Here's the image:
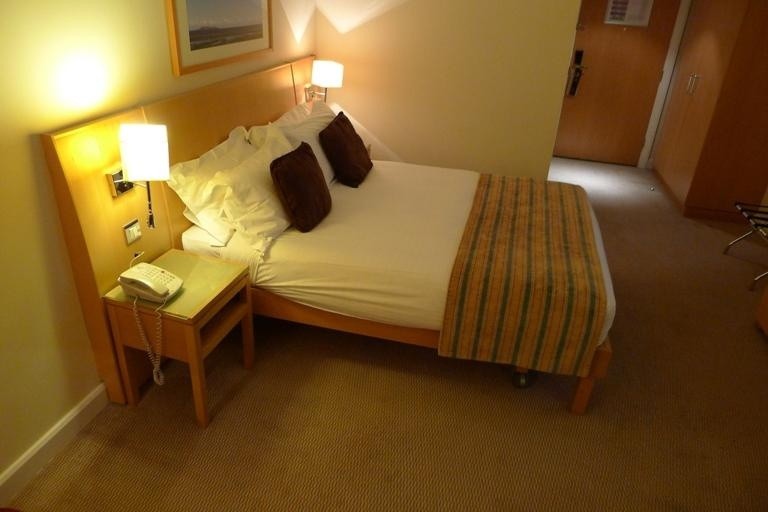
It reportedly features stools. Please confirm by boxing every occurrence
[724,201,768,291]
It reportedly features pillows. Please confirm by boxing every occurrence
[247,100,310,149]
[166,126,262,246]
[318,111,374,188]
[270,141,332,233]
[278,103,370,186]
[201,122,296,255]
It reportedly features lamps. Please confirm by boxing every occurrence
[118,122,170,229]
[311,60,344,102]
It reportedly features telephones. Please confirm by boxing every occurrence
[117,261,185,307]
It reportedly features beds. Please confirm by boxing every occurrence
[40,55,617,415]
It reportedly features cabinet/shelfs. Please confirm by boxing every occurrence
[647,0,768,213]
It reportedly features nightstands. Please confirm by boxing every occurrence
[101,247,256,428]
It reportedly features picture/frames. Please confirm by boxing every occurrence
[164,0,273,79]
